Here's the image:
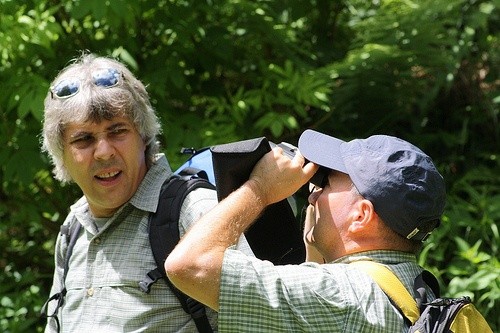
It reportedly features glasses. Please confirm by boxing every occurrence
[49,68,126,99]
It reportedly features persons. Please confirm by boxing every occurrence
[165,129,445,333]
[40,54,257,333]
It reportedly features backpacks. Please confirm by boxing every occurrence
[348,260,494,333]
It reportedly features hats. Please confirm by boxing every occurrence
[298,129,446,244]
